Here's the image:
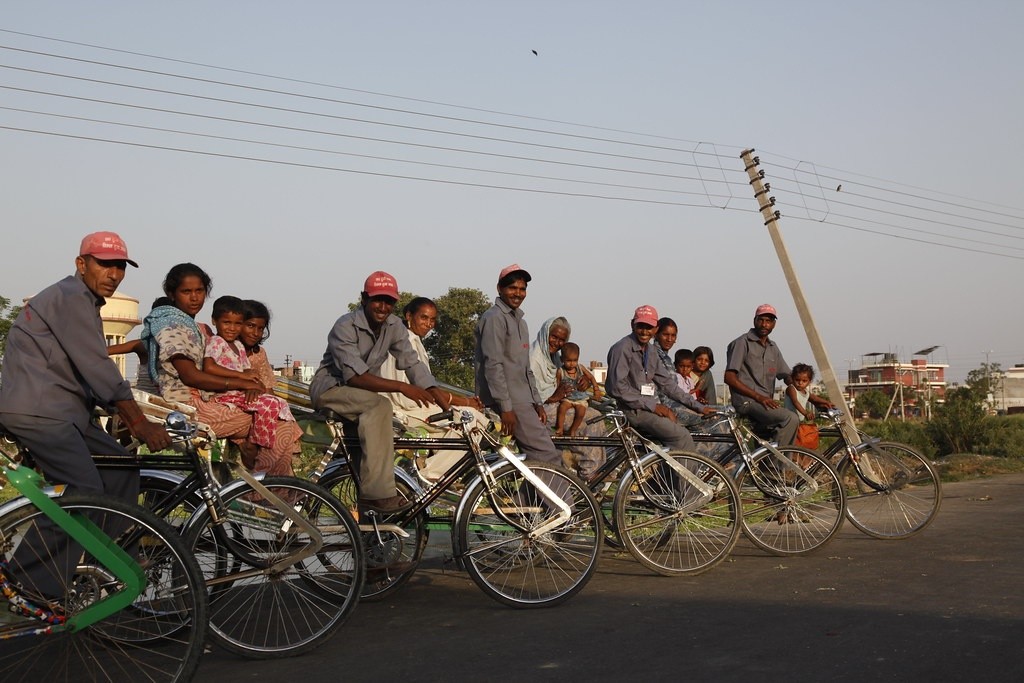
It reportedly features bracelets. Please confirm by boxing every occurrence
[448,393,452,404]
[593,386,600,392]
[464,397,469,405]
[225,377,228,388]
[447,406,453,411]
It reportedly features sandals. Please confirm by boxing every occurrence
[362,555,412,571]
[357,495,414,514]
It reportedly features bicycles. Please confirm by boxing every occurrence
[0,448,209,683]
[0,396,943,664]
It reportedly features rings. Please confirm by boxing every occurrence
[565,394,566,397]
[160,440,167,443]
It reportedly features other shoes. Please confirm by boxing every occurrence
[777,507,815,525]
[760,466,793,484]
[558,508,593,542]
[9,588,67,625]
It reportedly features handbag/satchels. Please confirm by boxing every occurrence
[793,419,819,450]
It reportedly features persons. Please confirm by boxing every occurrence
[474,264,622,532]
[605,305,717,516]
[0,230,304,622]
[309,271,462,584]
[724,304,835,525]
[377,297,489,492]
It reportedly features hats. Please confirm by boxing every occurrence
[756,303,778,321]
[80,232,139,269]
[364,271,401,301]
[498,264,532,282]
[633,305,659,327]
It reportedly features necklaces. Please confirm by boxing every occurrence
[566,369,577,378]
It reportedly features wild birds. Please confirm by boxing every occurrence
[532,50,537,56]
[837,185,842,192]
